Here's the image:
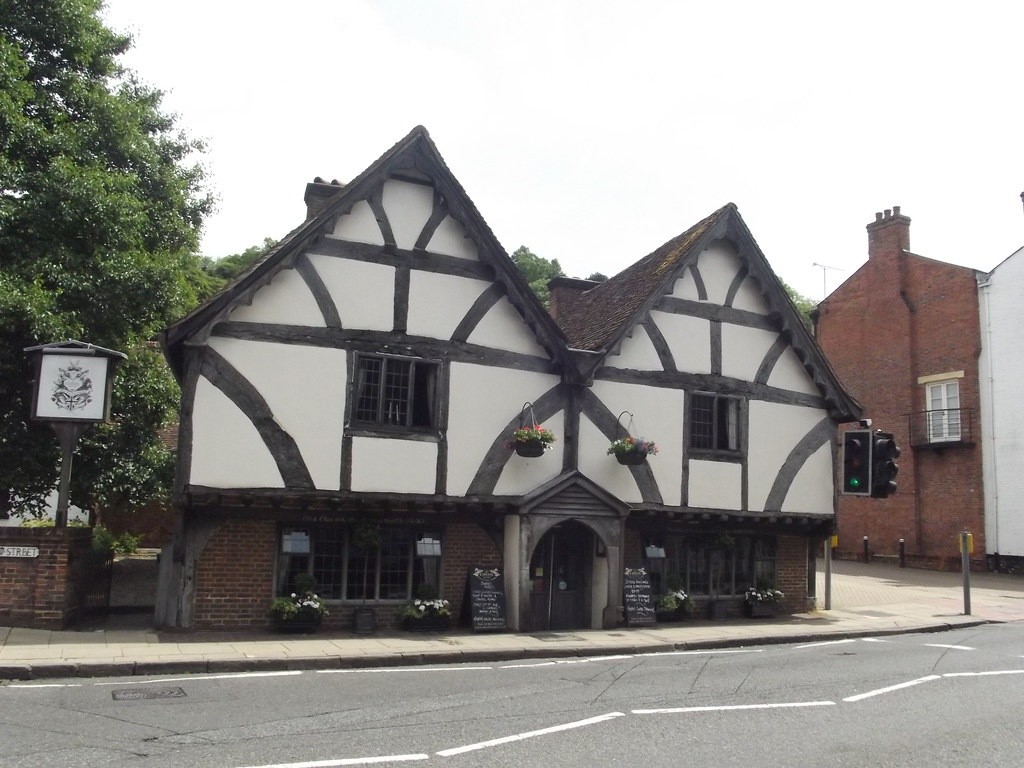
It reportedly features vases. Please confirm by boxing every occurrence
[750,603,779,616]
[613,450,647,465]
[273,617,321,632]
[405,618,449,632]
[515,438,547,457]
[657,612,684,622]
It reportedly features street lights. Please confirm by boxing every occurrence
[812,262,842,301]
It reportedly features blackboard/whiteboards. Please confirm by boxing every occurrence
[468,563,508,632]
[623,568,656,628]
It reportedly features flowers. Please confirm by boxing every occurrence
[268,592,330,618]
[745,587,785,606]
[606,437,659,457]
[505,424,556,451]
[653,589,693,612]
[400,599,452,619]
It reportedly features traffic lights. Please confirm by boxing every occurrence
[842,430,873,496]
[874,433,901,502]
